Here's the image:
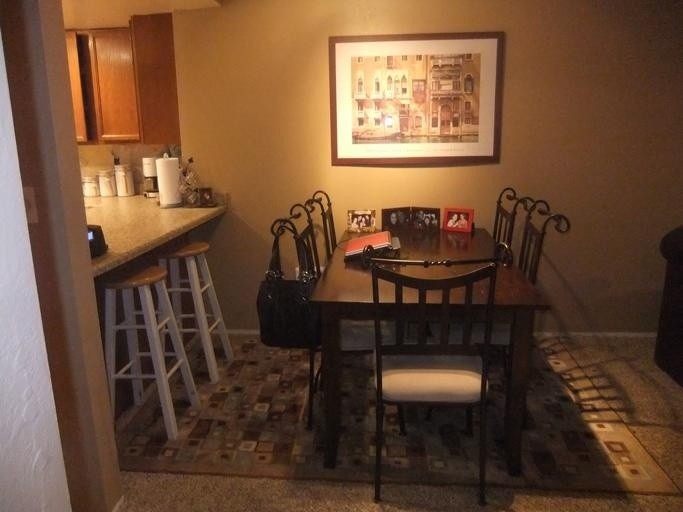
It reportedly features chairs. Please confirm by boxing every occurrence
[408,200,570,419]
[493,188,536,247]
[362,240,512,507]
[271,204,406,431]
[305,190,337,261]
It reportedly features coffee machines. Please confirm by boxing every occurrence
[142,157,160,198]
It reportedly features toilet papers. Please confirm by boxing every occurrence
[154,156,182,206]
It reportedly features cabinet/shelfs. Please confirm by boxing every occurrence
[64,13,181,143]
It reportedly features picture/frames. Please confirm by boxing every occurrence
[382,206,410,237]
[329,31,503,165]
[411,207,440,234]
[443,207,475,233]
[346,209,376,233]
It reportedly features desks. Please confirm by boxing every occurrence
[310,229,552,476]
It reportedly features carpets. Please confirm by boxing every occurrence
[114,334,683,512]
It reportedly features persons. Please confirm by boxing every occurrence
[414,210,438,232]
[447,213,468,228]
[384,211,409,228]
[351,215,371,228]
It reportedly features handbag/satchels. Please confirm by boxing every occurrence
[257,273,322,352]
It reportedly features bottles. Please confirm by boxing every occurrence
[82,163,135,197]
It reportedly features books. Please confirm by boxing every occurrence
[345,230,393,256]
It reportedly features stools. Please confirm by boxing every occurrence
[158,241,234,383]
[104,265,203,441]
